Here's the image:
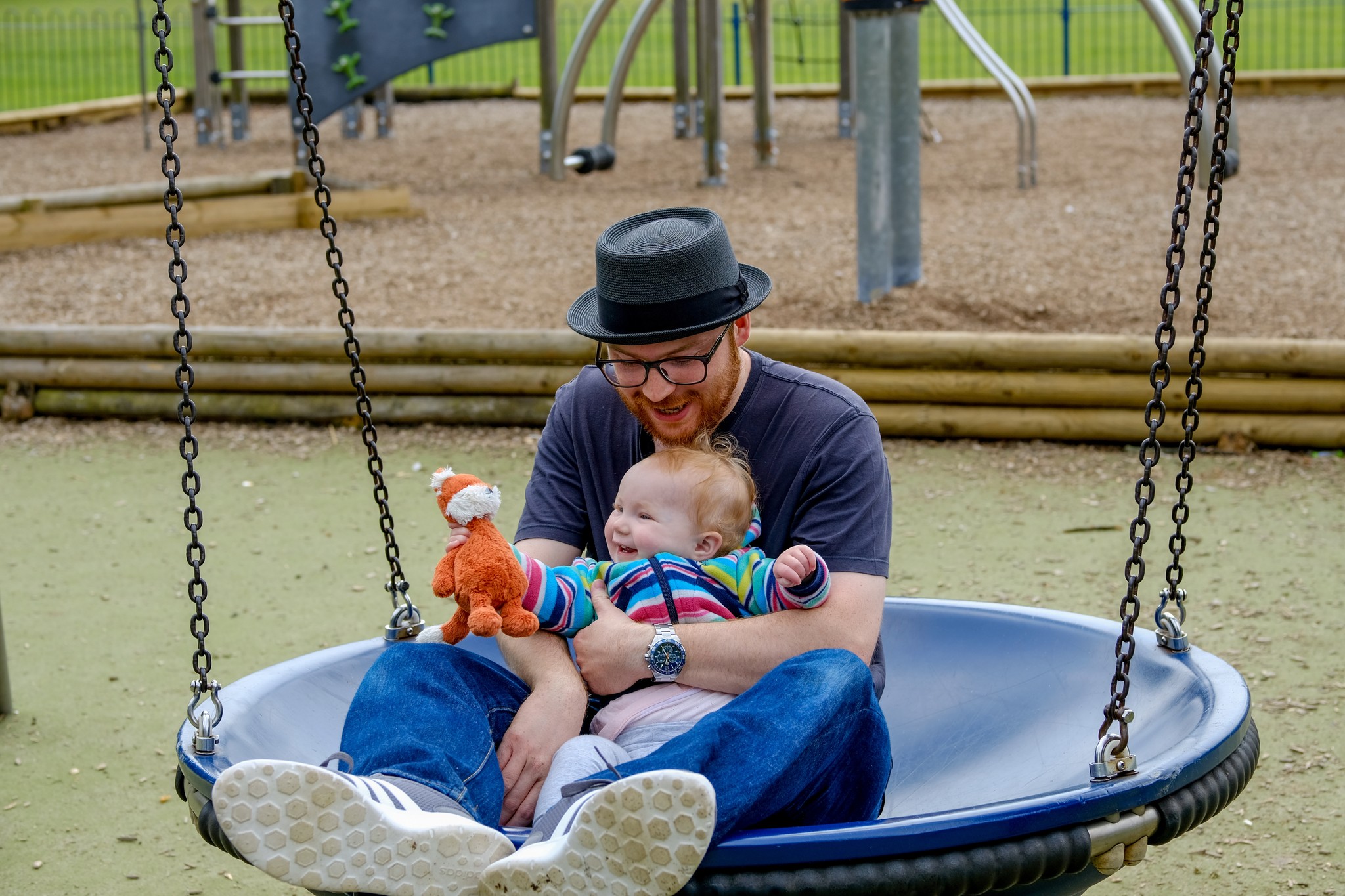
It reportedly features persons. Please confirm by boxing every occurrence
[212,222,896,896]
[444,423,831,835]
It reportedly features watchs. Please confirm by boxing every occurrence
[643,623,686,682]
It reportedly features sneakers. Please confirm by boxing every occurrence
[211,751,516,896]
[478,746,718,896]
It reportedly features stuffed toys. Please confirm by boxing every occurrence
[414,464,540,645]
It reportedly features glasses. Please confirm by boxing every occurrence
[594,320,733,388]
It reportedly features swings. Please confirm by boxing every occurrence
[138,0,1261,894]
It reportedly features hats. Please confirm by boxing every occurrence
[566,208,772,345]
